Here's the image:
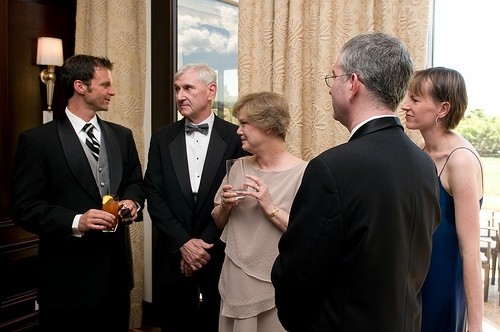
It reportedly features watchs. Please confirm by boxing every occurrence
[267,206,280,219]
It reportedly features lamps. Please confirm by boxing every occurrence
[36,37,64,124]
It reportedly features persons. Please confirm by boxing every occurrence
[271,32,441,332]
[18,54,145,332]
[402,65,485,332]
[211,91,310,331]
[144,64,253,331]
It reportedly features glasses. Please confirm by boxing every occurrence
[324,74,352,87]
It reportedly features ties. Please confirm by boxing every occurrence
[82,123,100,161]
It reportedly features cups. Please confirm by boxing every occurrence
[102,194,118,232]
[226,159,248,203]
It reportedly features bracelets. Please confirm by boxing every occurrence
[133,200,141,213]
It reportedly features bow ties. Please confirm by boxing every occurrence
[184,122,209,136]
